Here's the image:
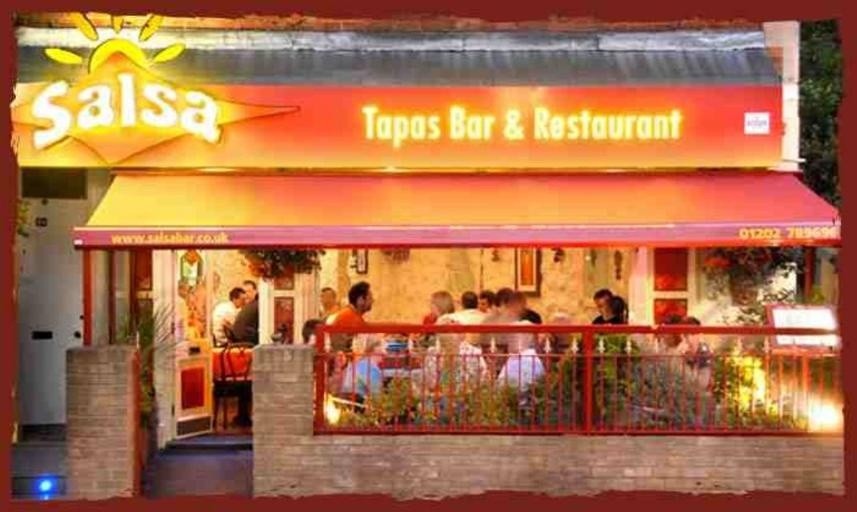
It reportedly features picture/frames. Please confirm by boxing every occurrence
[513,246,540,297]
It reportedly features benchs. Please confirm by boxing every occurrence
[762,301,841,397]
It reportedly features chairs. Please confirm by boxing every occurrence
[212,342,253,429]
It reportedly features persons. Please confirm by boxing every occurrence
[211,281,258,424]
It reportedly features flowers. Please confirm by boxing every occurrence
[700,246,807,302]
[242,247,328,282]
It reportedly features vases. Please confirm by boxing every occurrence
[248,262,271,277]
[729,278,758,305]
